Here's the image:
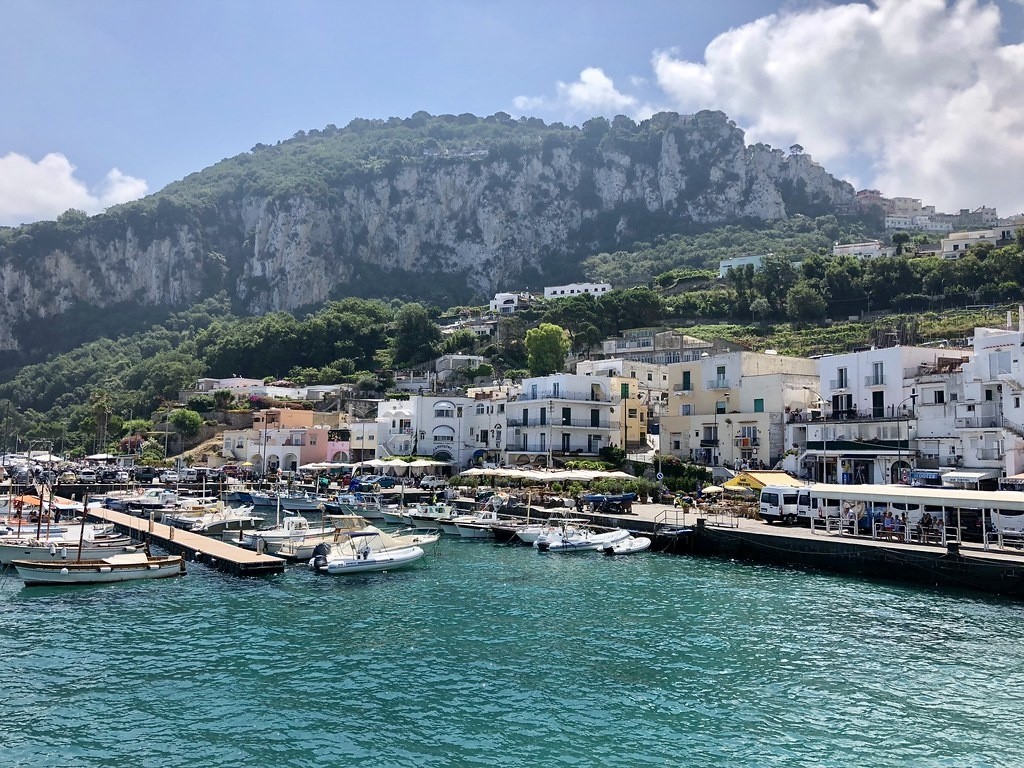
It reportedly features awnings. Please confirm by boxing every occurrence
[940,467,1002,483]
[1000,472,1024,490]
[910,471,939,479]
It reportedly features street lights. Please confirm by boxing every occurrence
[128,407,133,453]
[103,411,109,453]
[162,403,169,464]
[351,406,378,477]
[550,418,567,470]
[803,385,845,484]
[714,393,730,467]
[897,393,919,483]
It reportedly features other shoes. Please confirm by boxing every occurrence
[888,538,893,541]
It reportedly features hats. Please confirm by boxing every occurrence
[882,512,887,514]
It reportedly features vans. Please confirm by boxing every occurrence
[177,468,198,483]
[796,485,840,530]
[841,473,1024,549]
[217,464,239,478]
[758,483,800,526]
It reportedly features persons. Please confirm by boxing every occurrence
[878,511,944,546]
[733,457,765,475]
[14,509,62,523]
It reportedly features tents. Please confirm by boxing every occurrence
[297,459,454,479]
[719,470,805,489]
[459,468,637,491]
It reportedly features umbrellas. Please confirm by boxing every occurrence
[242,461,255,472]
[723,485,746,503]
[701,485,725,497]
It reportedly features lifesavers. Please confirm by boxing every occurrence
[138,488,144,495]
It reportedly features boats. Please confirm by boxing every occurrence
[0,458,592,549]
[313,530,426,576]
[10,487,183,587]
[536,528,631,555]
[604,536,651,555]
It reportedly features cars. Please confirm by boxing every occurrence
[36,471,57,484]
[116,471,131,484]
[95,469,117,484]
[74,470,96,484]
[11,470,34,485]
[58,471,77,485]
[159,470,179,483]
[366,475,396,489]
[360,474,381,483]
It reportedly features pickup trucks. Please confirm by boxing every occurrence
[133,466,155,484]
[419,476,446,491]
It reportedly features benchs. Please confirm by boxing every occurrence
[986,530,1024,550]
[812,517,959,546]
